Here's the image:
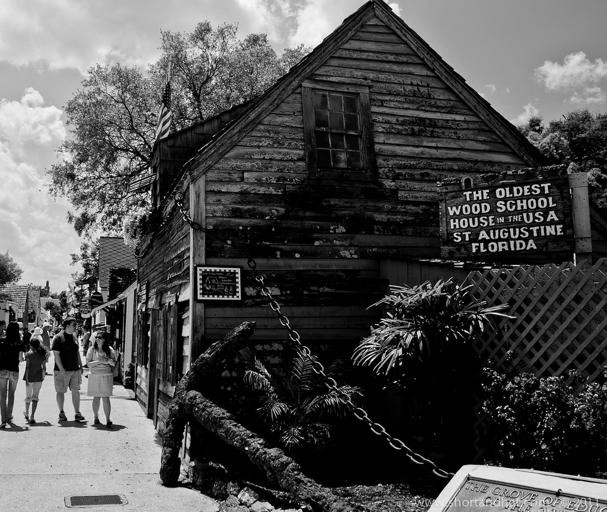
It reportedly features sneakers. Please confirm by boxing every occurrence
[75,412,85,420]
[0,412,36,428]
[59,411,67,421]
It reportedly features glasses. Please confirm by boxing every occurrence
[95,336,102,338]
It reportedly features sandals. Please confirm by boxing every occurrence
[94,419,114,429]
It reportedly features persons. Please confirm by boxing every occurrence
[0,319,117,431]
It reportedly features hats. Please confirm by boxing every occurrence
[42,322,52,331]
[35,327,43,334]
